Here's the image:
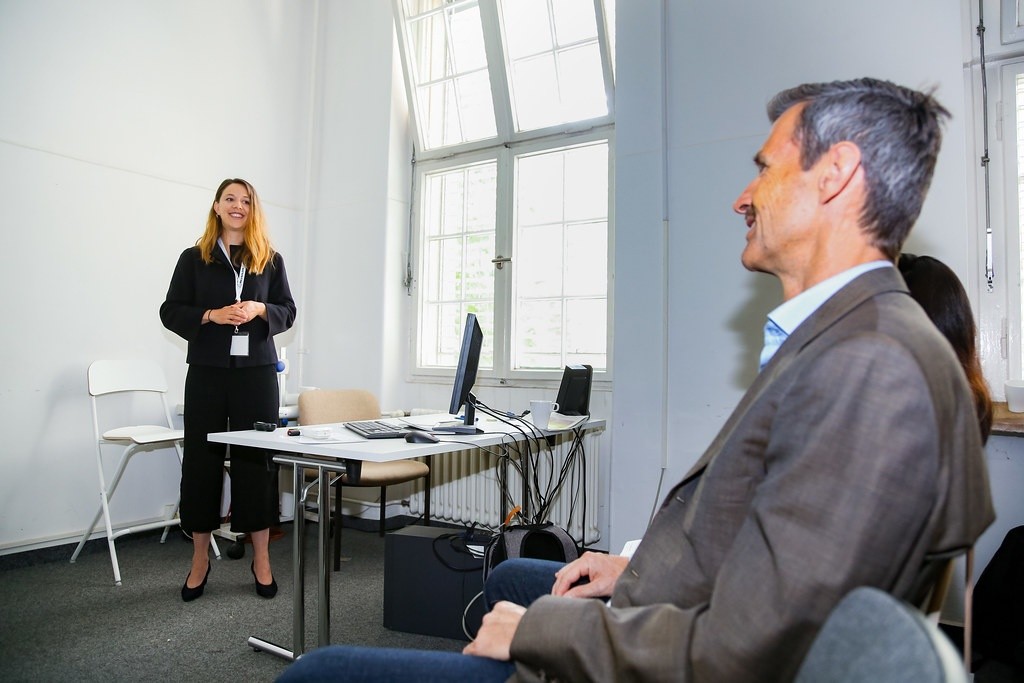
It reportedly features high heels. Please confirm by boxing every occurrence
[182,557,211,602]
[250,560,279,599]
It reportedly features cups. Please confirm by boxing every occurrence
[1004,379,1024,413]
[530,400,559,431]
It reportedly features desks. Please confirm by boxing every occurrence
[205,402,613,661]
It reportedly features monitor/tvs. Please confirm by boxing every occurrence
[432,313,484,434]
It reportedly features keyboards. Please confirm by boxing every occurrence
[344,421,412,440]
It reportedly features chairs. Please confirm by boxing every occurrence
[297,388,437,576]
[67,355,222,586]
[749,585,986,683]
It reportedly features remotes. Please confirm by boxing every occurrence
[253,421,276,431]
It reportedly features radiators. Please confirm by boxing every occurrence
[409,408,601,545]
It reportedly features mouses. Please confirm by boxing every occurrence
[405,431,440,443]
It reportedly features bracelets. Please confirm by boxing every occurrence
[208,308,213,322]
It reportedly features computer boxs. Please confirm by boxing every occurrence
[382,525,504,641]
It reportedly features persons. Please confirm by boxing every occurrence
[274,78,999,683]
[898,253,994,448]
[159,178,298,603]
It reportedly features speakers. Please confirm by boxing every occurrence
[554,364,594,417]
